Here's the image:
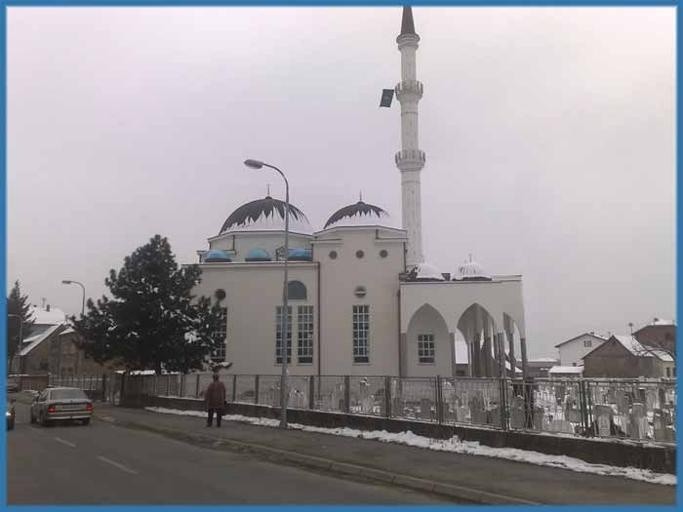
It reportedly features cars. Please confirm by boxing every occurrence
[6,396,15,430]
[30,385,94,428]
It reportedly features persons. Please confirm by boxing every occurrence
[203,372,227,428]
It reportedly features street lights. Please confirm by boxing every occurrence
[244,159,289,425]
[8,312,22,390]
[64,280,84,377]
